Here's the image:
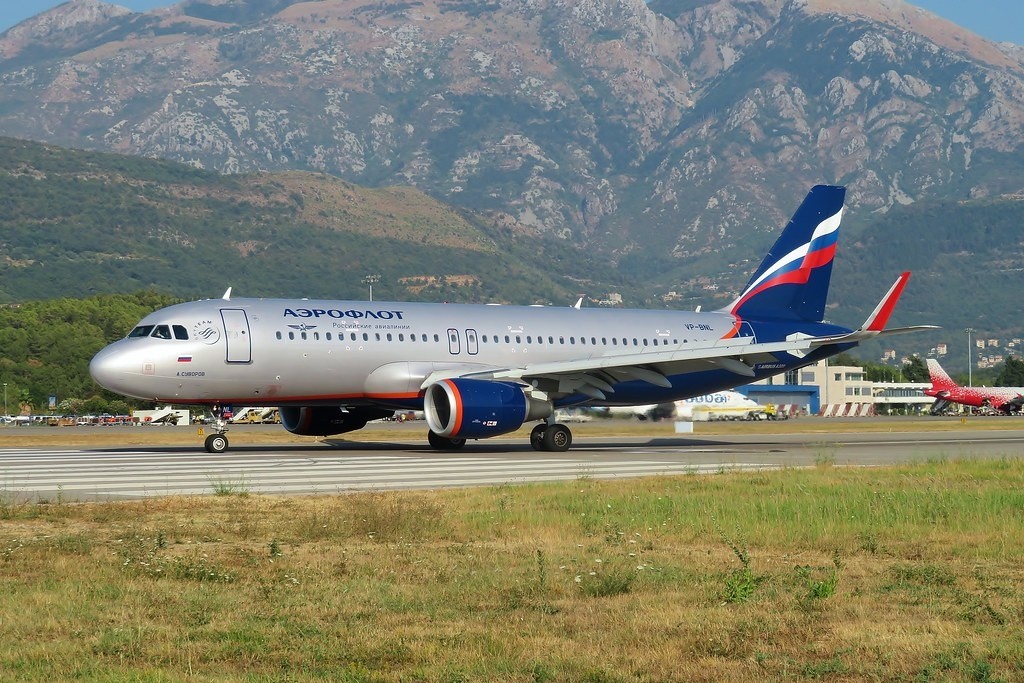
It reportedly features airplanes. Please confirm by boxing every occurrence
[90,185,942,451]
[924,359,1024,416]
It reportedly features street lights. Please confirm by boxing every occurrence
[3,382,8,416]
[362,273,381,301]
[963,328,977,415]
